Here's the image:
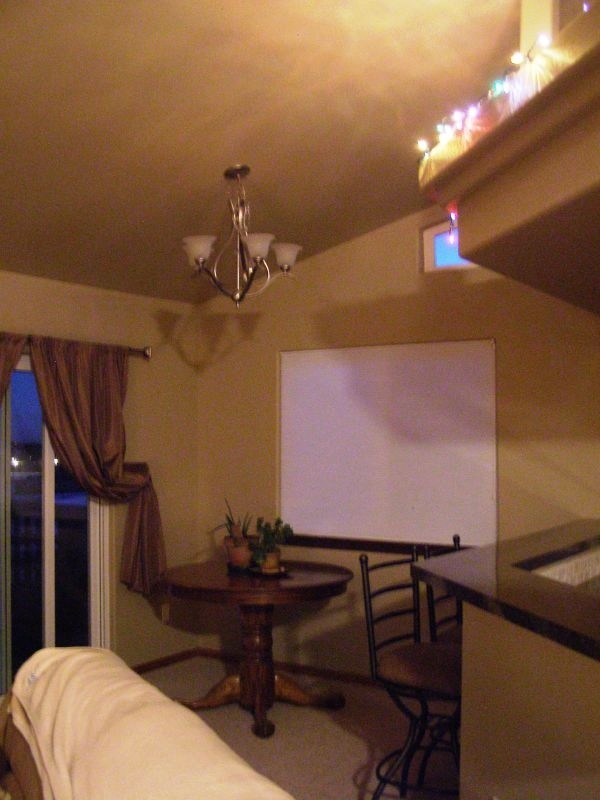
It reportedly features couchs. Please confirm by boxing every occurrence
[0,647,294,800]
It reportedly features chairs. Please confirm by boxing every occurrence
[359,534,462,800]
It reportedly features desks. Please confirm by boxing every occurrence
[166,560,355,738]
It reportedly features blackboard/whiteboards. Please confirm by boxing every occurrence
[279,337,498,548]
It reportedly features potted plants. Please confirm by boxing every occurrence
[209,498,294,576]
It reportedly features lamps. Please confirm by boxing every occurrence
[182,163,302,310]
